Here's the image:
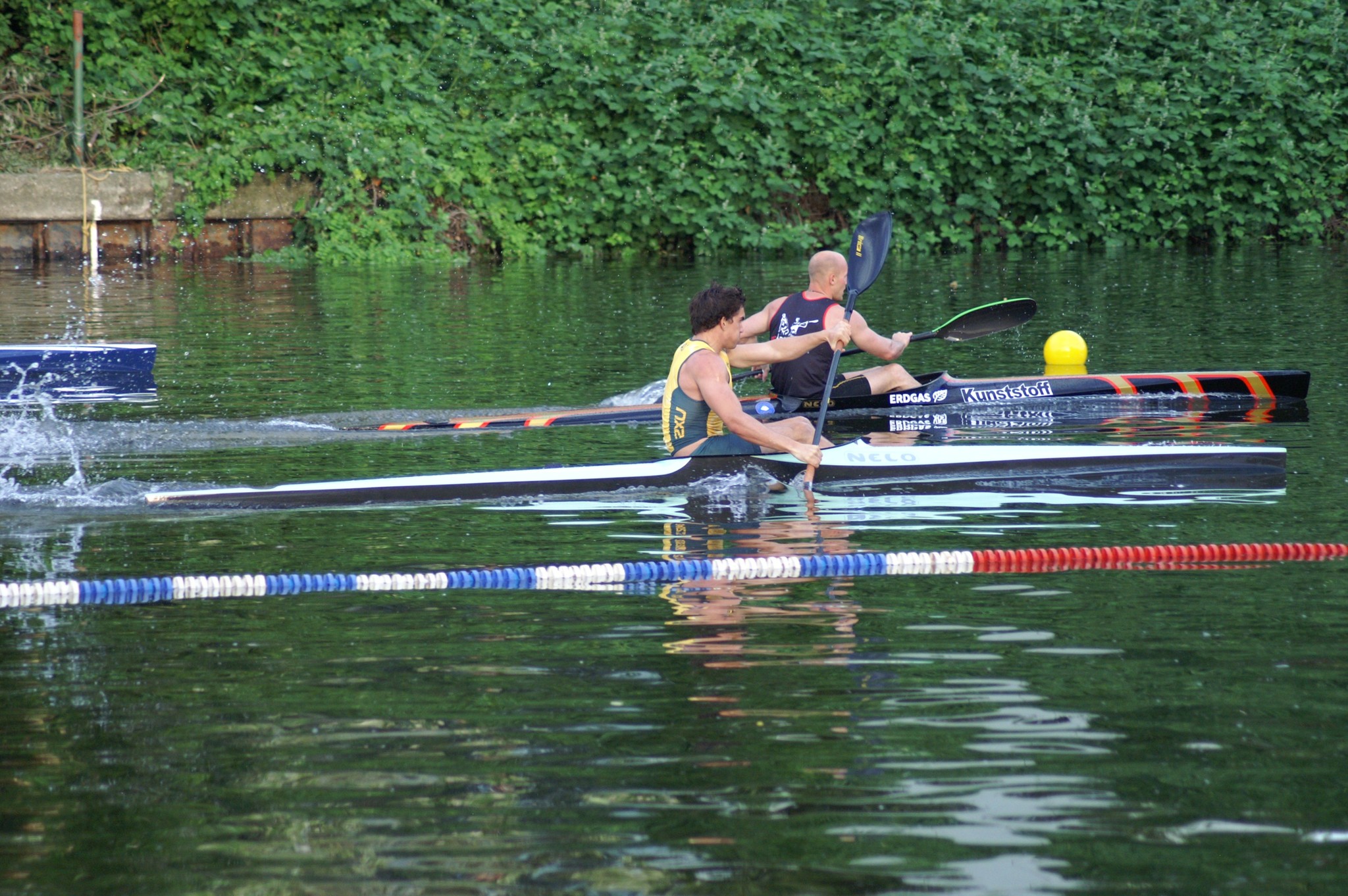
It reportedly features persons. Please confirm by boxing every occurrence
[733,250,925,401]
[663,280,833,467]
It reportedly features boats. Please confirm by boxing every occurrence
[144,443,1288,505]
[0,342,160,382]
[340,365,1311,433]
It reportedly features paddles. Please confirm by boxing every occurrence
[729,297,1038,382]
[800,207,895,500]
